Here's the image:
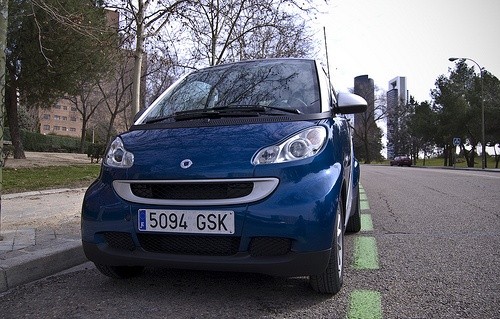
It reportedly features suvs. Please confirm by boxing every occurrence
[390,156,412,167]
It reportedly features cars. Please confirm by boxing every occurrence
[80,57,368,295]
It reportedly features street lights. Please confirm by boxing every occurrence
[449,57,485,169]
